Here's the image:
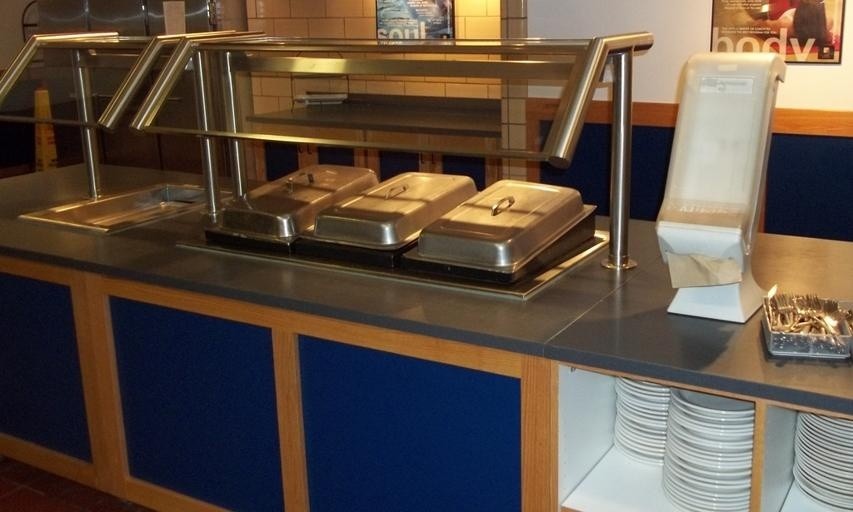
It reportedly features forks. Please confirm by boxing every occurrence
[768,284,850,348]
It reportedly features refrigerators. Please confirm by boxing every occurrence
[37,0,258,180]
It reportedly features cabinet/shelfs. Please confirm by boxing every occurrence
[536,357,853,510]
[366,129,488,193]
[0,246,109,494]
[79,272,560,510]
[251,119,366,184]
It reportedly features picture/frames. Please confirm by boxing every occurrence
[709,0,845,65]
[375,0,456,40]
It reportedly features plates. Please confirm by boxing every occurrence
[792,411,853,510]
[614,375,755,512]
